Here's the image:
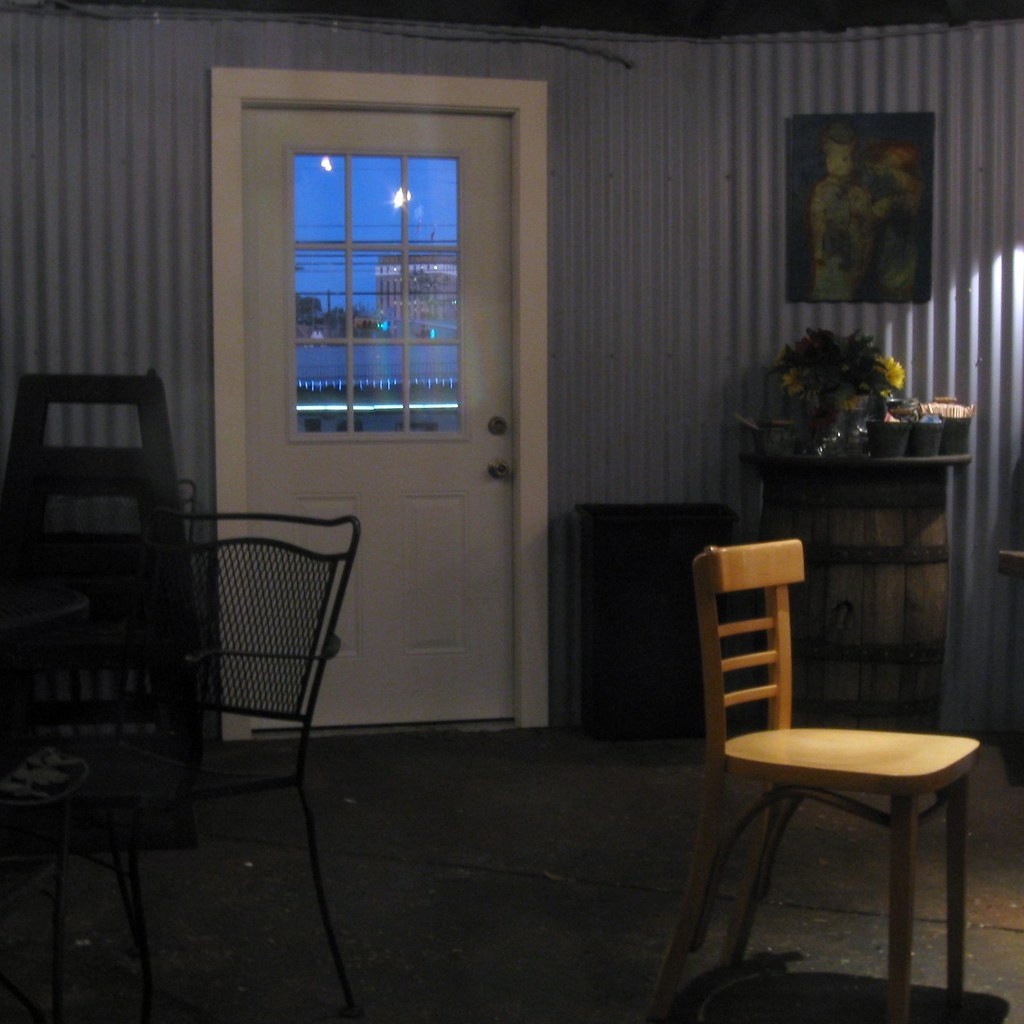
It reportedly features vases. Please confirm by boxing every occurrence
[803,395,871,455]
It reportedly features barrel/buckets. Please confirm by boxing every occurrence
[758,477,951,733]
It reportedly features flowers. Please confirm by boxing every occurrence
[769,327,907,407]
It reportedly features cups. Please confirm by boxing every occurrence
[941,417,970,455]
[910,424,945,457]
[864,421,909,459]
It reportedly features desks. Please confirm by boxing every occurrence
[738,453,972,728]
[0,579,92,773]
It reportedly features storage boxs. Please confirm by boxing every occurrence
[577,501,740,741]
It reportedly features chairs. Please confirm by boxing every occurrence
[0,509,361,1024]
[637,537,981,1024]
[0,370,199,801]
